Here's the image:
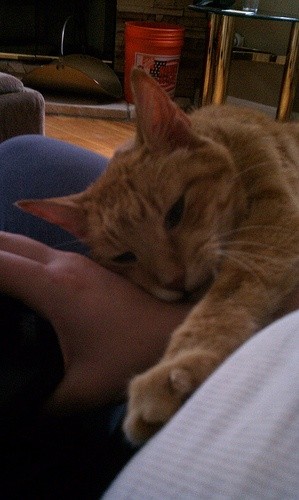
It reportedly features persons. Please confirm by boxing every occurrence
[0,135,299,500]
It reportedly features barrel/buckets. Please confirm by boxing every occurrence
[123,22,183,104]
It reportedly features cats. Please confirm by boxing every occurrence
[14,66,299,448]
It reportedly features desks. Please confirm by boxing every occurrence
[189,4,299,121]
